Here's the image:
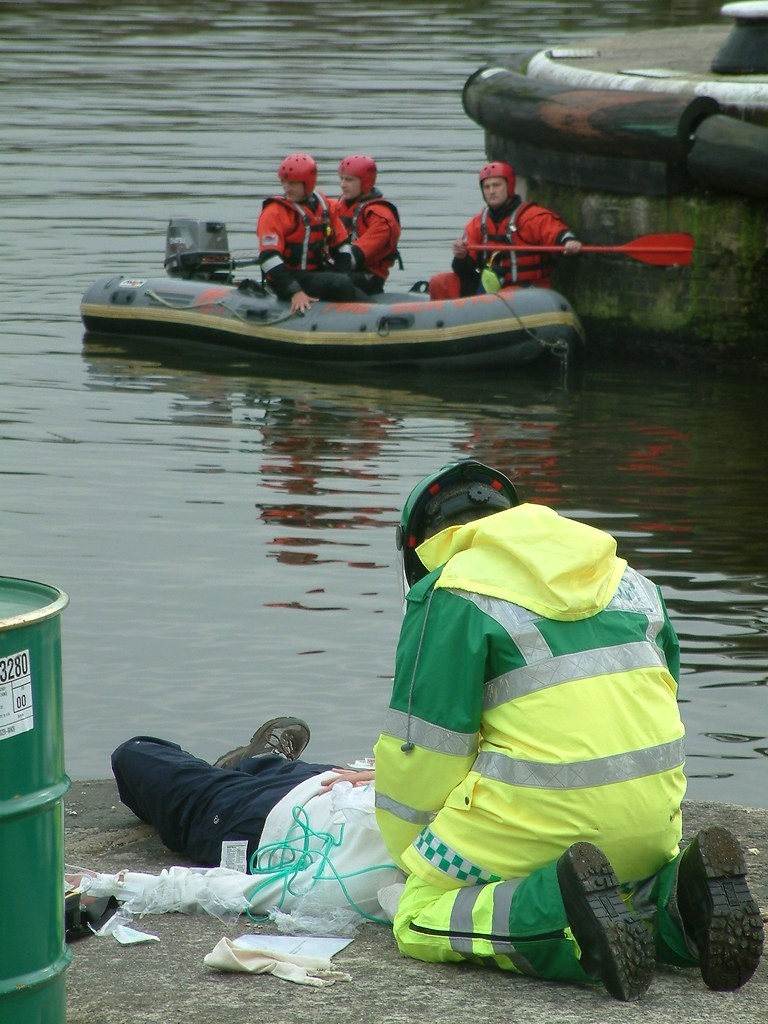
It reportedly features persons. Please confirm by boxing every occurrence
[65,715,406,923]
[257,153,401,315]
[375,456,764,1002]
[429,162,582,300]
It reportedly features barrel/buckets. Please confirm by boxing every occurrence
[0,575,74,1024]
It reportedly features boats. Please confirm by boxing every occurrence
[76,217,588,372]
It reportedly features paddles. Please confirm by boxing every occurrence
[465,233,693,267]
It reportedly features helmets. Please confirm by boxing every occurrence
[480,162,516,204]
[395,458,520,589]
[339,155,377,193]
[278,154,316,195]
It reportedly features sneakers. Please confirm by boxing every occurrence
[212,716,310,771]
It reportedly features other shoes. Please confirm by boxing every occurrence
[556,841,655,1001]
[678,828,764,992]
